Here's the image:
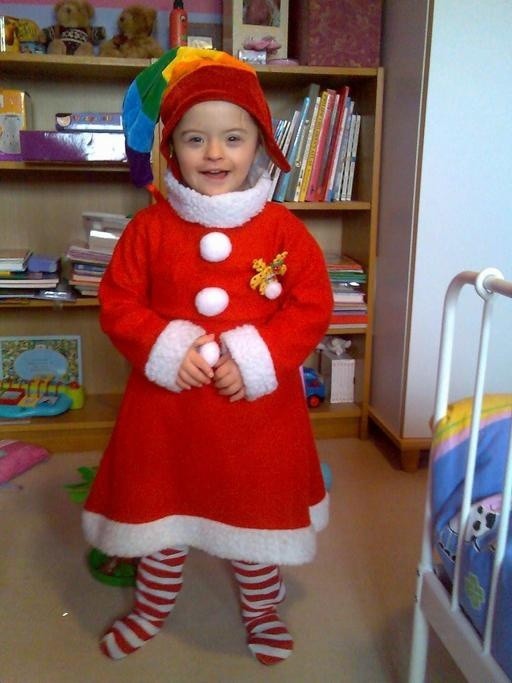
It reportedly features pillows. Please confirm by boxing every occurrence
[0,438,49,484]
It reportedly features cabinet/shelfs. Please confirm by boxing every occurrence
[369,1,512,474]
[0,49,384,455]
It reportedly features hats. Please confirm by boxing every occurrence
[122,46,290,187]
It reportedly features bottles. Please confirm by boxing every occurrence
[169,0,188,51]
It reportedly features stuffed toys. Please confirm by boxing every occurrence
[38,0,105,57]
[100,4,165,58]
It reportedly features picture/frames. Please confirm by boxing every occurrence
[0,335,83,385]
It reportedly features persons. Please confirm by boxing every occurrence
[79,46,333,667]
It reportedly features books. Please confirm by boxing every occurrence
[0,246,78,303]
[319,254,368,330]
[64,210,135,296]
[259,83,360,203]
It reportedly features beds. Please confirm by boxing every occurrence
[408,266,512,683]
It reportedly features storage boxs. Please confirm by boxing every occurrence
[0,88,31,159]
[321,352,355,404]
[290,0,382,67]
[19,129,129,164]
